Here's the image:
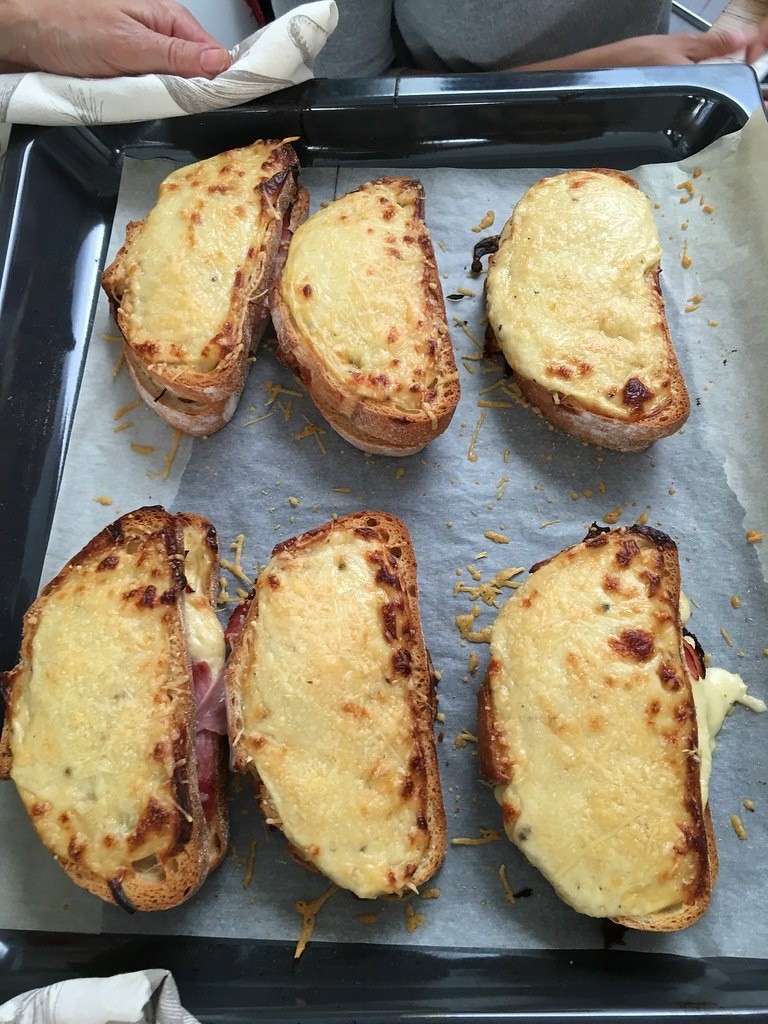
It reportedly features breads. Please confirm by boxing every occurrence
[100,137,310,437]
[0,502,232,914]
[483,523,718,933]
[488,167,692,454]
[268,176,461,457]
[220,510,445,902]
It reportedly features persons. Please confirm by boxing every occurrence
[0,0,768,79]
